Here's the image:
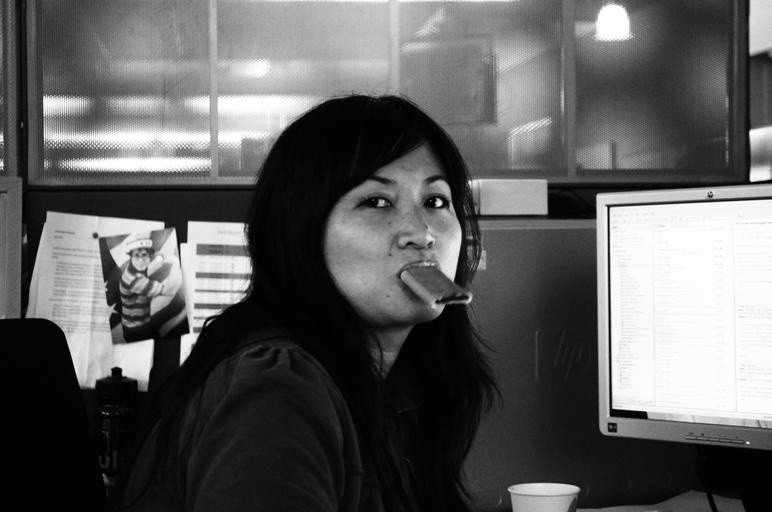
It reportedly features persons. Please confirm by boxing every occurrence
[120,88,509,508]
[116,237,181,343]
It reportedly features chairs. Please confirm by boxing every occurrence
[1,318,82,512]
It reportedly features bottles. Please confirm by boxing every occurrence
[94,368,139,507]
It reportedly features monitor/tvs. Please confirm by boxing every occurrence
[596,184,772,451]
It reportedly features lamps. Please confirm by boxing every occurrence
[594,1,632,42]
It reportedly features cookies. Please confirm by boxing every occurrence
[400,266,473,309]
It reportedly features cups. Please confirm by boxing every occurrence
[507,483,579,512]
